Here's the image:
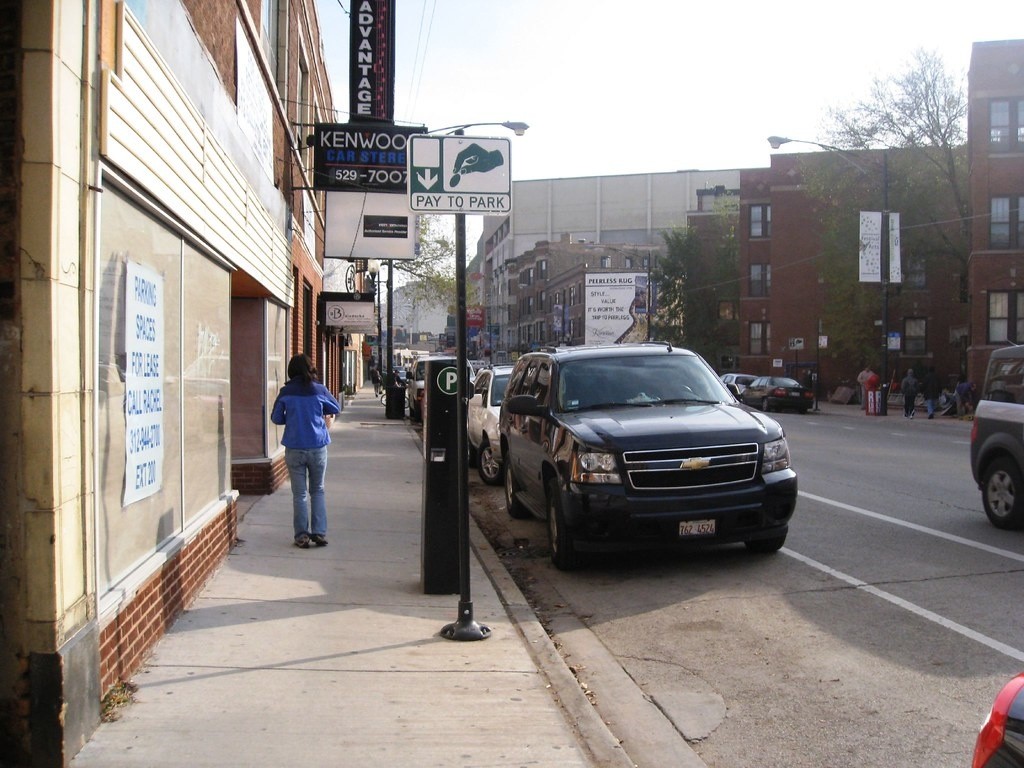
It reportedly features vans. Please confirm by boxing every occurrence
[407,355,474,424]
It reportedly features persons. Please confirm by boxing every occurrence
[806,369,813,388]
[953,375,968,417]
[394,369,408,387]
[271,354,340,548]
[857,365,875,410]
[372,371,383,398]
[922,368,939,419]
[901,369,919,419]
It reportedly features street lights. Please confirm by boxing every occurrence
[441,126,528,643]
[585,243,652,341]
[368,258,381,396]
[768,134,892,416]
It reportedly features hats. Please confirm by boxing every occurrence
[908,369,914,374]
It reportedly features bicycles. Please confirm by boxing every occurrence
[381,382,411,410]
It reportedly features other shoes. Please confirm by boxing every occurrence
[903,414,913,419]
[928,413,934,419]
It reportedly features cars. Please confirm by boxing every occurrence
[468,362,515,487]
[741,375,814,415]
[719,373,759,402]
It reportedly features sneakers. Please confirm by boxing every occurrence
[294,535,329,549]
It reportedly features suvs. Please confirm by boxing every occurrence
[499,341,797,574]
[969,343,1024,530]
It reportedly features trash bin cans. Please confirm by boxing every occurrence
[864,376,888,416]
[386,387,406,419]
